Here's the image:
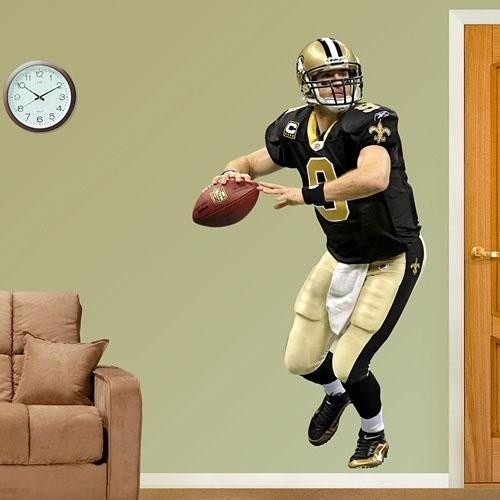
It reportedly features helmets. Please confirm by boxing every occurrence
[296,38,363,113]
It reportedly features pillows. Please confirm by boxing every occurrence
[13,331,109,406]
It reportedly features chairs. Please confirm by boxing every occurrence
[0,291,140,498]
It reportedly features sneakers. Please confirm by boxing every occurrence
[348,429,389,468]
[308,391,351,446]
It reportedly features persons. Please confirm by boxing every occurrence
[212,37,424,468]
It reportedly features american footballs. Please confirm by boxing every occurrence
[193,179,260,227]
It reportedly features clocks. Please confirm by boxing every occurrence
[5,60,77,131]
